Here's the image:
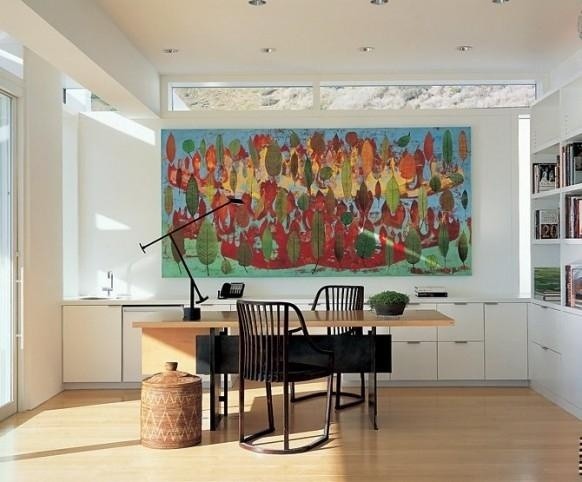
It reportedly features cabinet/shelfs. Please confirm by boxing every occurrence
[62,300,184,390]
[529,299,582,422]
[437,301,485,386]
[529,71,581,310]
[390,302,437,387]
[486,299,529,387]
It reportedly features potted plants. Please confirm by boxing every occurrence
[367,291,409,316]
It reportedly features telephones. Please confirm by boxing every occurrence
[217,282,245,299]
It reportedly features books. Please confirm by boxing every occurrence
[562,142,582,187]
[534,266,561,293]
[564,193,582,239]
[532,154,561,194]
[533,208,561,240]
[414,286,448,297]
[565,264,582,309]
[533,293,561,301]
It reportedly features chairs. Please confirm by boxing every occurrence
[234,300,332,454]
[291,285,365,410]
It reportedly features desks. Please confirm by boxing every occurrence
[131,310,454,431]
